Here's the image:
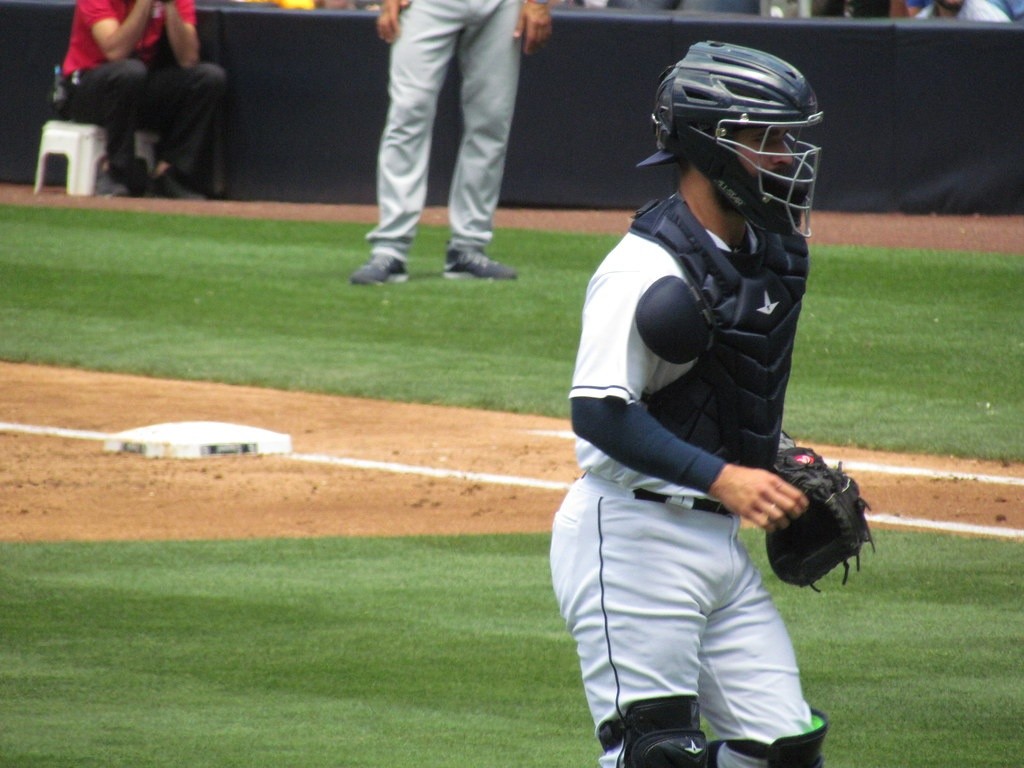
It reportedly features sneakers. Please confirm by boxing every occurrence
[444,251,516,280]
[352,253,407,285]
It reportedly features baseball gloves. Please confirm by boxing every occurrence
[764,446,871,590]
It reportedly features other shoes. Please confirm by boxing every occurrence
[149,166,204,199]
[112,177,130,195]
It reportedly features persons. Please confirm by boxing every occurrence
[549,42,829,768]
[60,0,226,201]
[350,0,553,285]
[915,0,1014,23]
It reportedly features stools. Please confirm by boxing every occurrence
[30,120,159,197]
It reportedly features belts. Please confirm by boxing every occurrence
[635,488,729,516]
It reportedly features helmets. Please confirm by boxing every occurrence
[637,40,823,239]
